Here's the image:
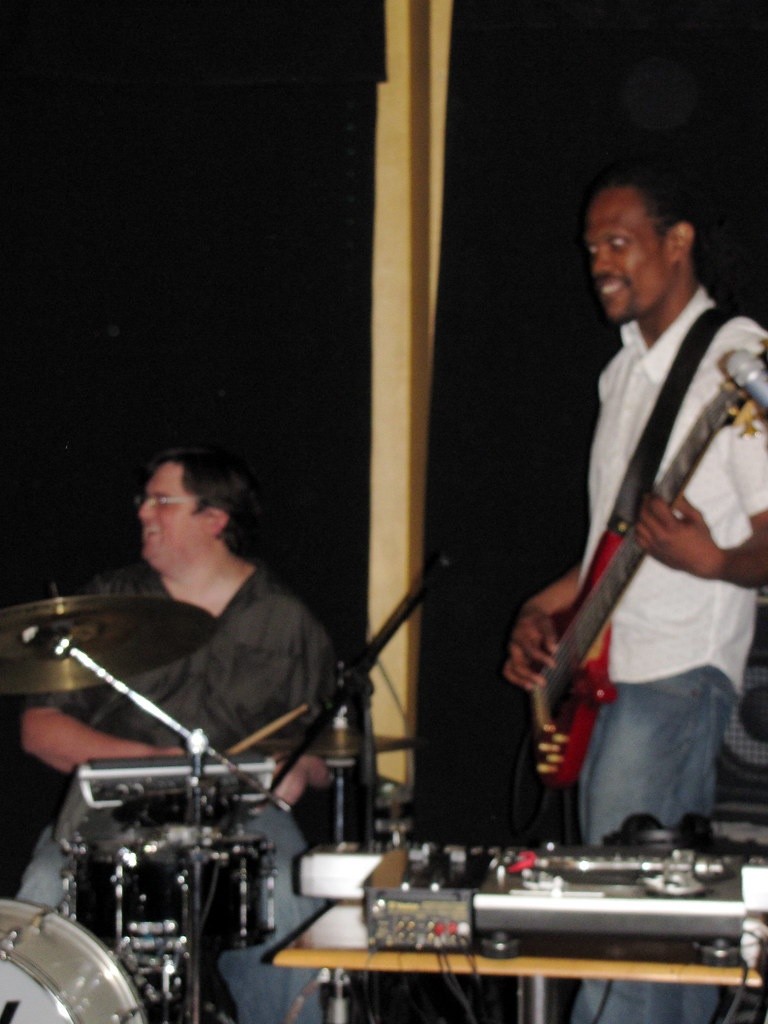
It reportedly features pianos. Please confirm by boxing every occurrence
[292,841,767,901]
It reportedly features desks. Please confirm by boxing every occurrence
[274,898,768,1024]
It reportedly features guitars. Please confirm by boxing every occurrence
[525,333,767,786]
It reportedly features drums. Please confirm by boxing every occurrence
[69,826,271,1024]
[0,894,151,1024]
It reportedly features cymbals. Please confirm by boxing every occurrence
[257,733,418,758]
[0,595,217,694]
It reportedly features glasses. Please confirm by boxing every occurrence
[134,494,195,507]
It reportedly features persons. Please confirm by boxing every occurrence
[16,443,336,1024]
[503,160,768,1024]
